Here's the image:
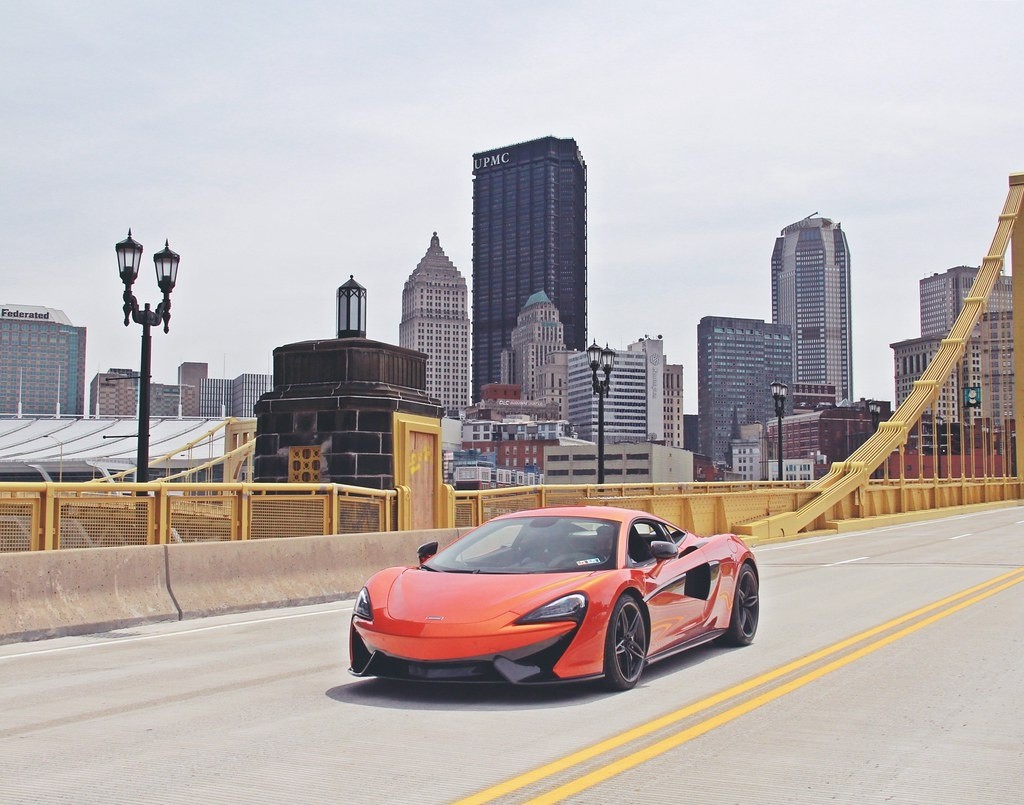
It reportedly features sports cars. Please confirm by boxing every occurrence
[347,503,761,690]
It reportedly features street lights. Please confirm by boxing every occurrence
[934,413,945,478]
[103,226,181,545]
[759,376,791,485]
[869,397,882,485]
[573,337,615,493]
[44,434,64,482]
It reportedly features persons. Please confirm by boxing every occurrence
[594,526,637,568]
